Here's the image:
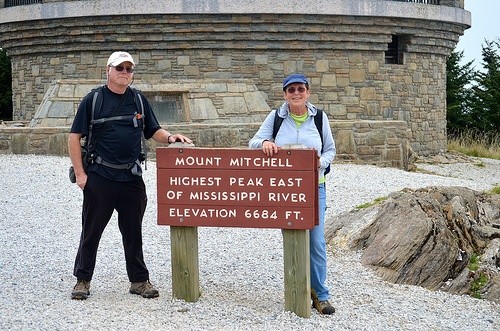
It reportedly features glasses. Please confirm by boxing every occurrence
[285,86,307,94]
[109,64,134,73]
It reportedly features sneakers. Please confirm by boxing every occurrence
[129,280,159,298]
[71,278,90,299]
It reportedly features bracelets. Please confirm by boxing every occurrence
[168,135,172,144]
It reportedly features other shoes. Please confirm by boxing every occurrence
[318,301,335,315]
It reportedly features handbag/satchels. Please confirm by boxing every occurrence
[69,149,88,183]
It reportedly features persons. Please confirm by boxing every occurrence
[248,74,336,314]
[68,51,193,300]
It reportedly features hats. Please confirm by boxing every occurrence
[283,74,309,89]
[107,51,136,67]
